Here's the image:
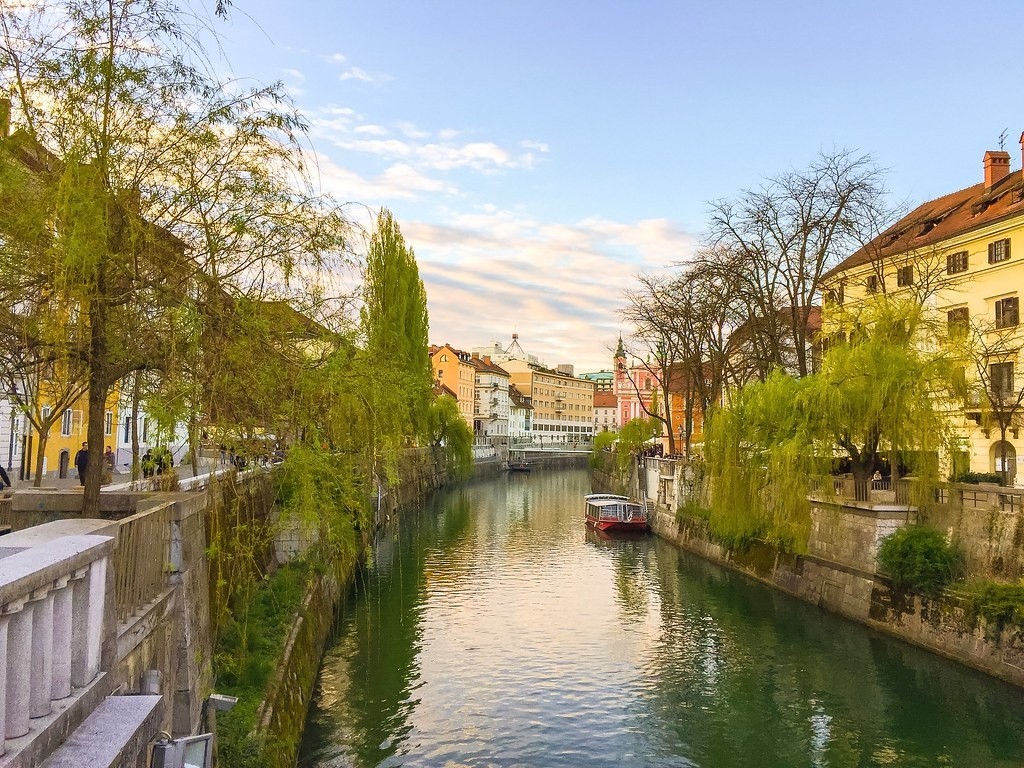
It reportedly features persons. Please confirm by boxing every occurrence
[104,446,116,472]
[142,448,174,478]
[218,440,275,465]
[874,470,882,481]
[74,442,88,486]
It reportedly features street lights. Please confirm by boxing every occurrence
[677,424,683,453]
[5,390,21,472]
[652,427,657,456]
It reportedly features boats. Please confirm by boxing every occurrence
[508,448,530,470]
[584,494,648,530]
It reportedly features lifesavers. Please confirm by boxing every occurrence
[520,465,524,469]
